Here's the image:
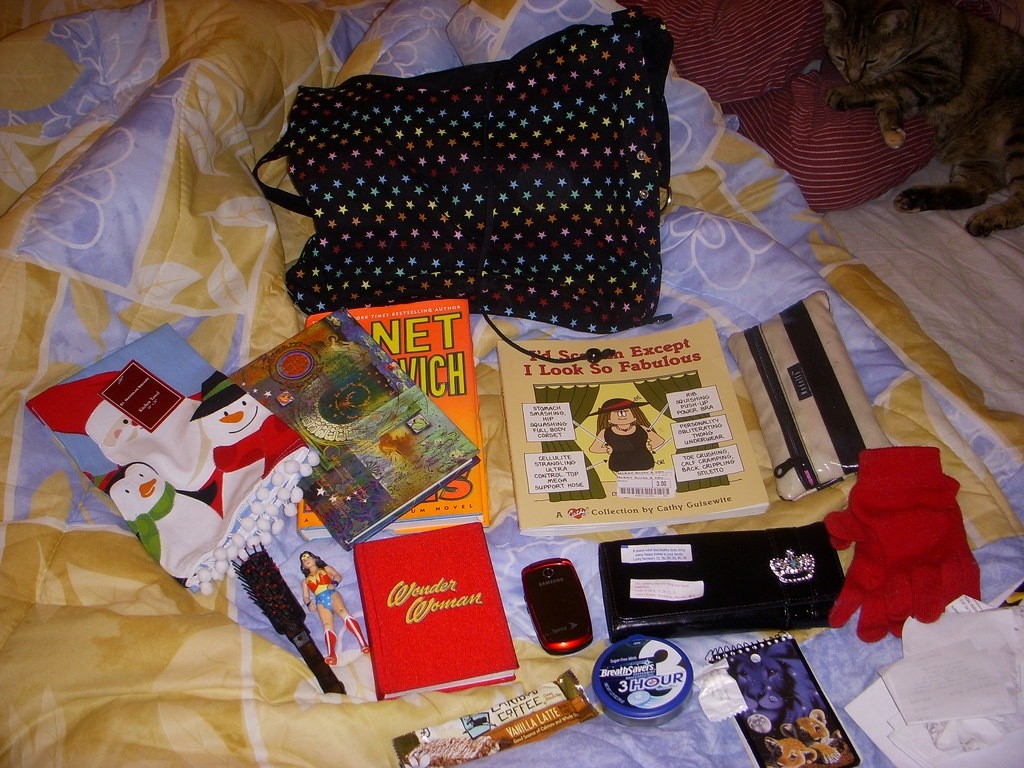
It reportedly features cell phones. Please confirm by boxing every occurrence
[522,557,593,655]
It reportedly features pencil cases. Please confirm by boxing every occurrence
[725,292,897,502]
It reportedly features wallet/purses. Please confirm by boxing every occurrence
[598,521,845,643]
[728,291,893,499]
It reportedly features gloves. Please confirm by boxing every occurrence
[824,446,981,644]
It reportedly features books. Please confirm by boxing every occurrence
[703,630,864,768]
[227,305,482,553]
[353,523,520,701]
[297,298,491,543]
[496,316,770,538]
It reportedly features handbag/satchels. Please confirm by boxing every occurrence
[253,6,672,363]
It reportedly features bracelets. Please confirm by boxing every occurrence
[331,580,338,587]
[306,602,310,606]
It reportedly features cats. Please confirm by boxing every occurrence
[821,0,1024,238]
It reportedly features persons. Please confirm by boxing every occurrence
[299,549,370,665]
